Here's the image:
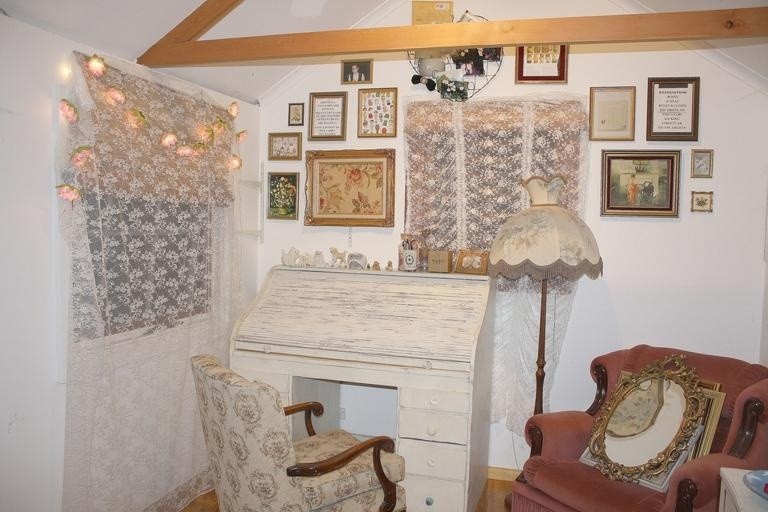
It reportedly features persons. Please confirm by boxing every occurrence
[627,174,638,204]
[348,65,366,81]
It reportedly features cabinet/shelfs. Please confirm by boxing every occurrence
[228,350,489,512]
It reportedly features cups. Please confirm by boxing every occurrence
[402,249,418,272]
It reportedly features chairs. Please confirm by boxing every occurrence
[190,354,406,512]
[504,344,768,512]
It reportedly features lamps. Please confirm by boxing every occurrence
[489,178,603,512]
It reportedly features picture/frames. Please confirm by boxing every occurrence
[455,251,488,275]
[601,151,681,216]
[691,191,713,212]
[589,87,636,141]
[515,44,568,84]
[265,58,398,228]
[691,149,713,178]
[579,351,728,494]
[646,77,700,141]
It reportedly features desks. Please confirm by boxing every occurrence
[719,466,768,512]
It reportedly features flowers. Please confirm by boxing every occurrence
[53,54,247,202]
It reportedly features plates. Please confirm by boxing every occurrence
[743,468,768,500]
